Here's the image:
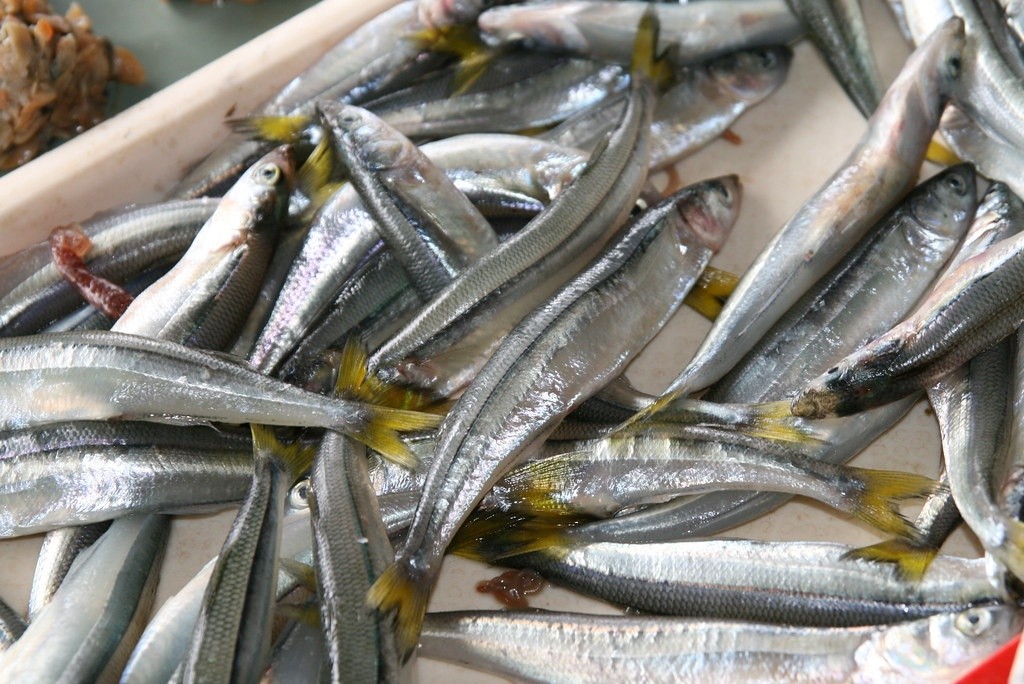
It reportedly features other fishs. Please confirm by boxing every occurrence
[0,0,1024,684]
